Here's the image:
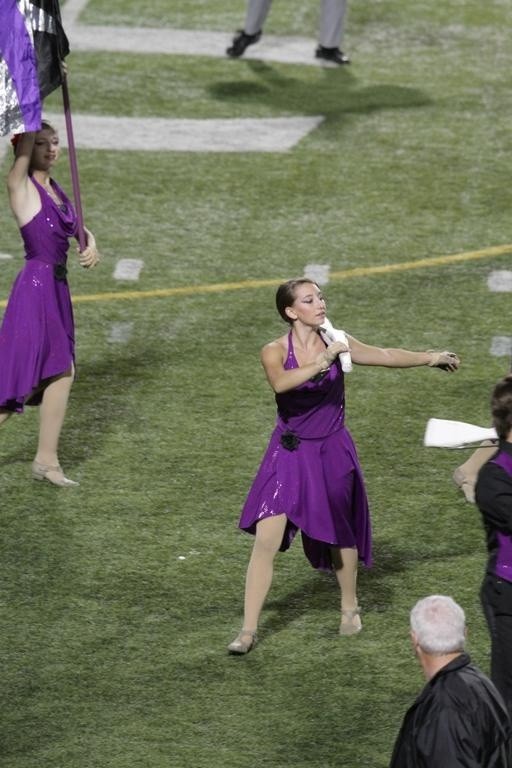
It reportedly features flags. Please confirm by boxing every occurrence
[1,1,76,137]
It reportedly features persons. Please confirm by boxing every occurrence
[385,595,511,767]
[1,118,99,486]
[221,278,459,655]
[472,376,512,710]
[217,0,354,64]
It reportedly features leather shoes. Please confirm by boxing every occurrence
[226,30,260,57]
[316,45,348,64]
[341,607,362,635]
[226,631,257,654]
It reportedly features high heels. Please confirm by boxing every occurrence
[32,460,78,488]
